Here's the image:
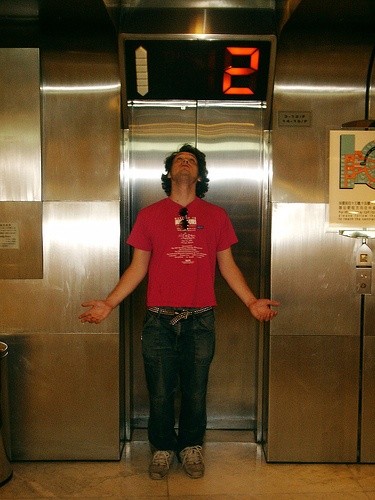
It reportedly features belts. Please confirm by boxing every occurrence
[148,306,213,326]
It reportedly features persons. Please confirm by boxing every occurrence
[78,144,280,480]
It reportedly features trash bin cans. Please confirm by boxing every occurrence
[0,341,14,487]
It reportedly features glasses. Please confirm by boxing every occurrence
[178,207,189,231]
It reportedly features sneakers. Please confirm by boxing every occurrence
[178,445,205,478]
[149,450,175,480]
